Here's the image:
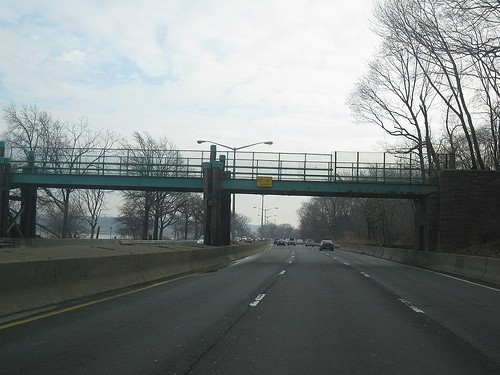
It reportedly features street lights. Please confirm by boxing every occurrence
[196,139,279,246]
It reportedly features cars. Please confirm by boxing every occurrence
[319,239,334,251]
[274,237,303,247]
[229,235,266,244]
[196,235,205,245]
[304,239,314,248]
